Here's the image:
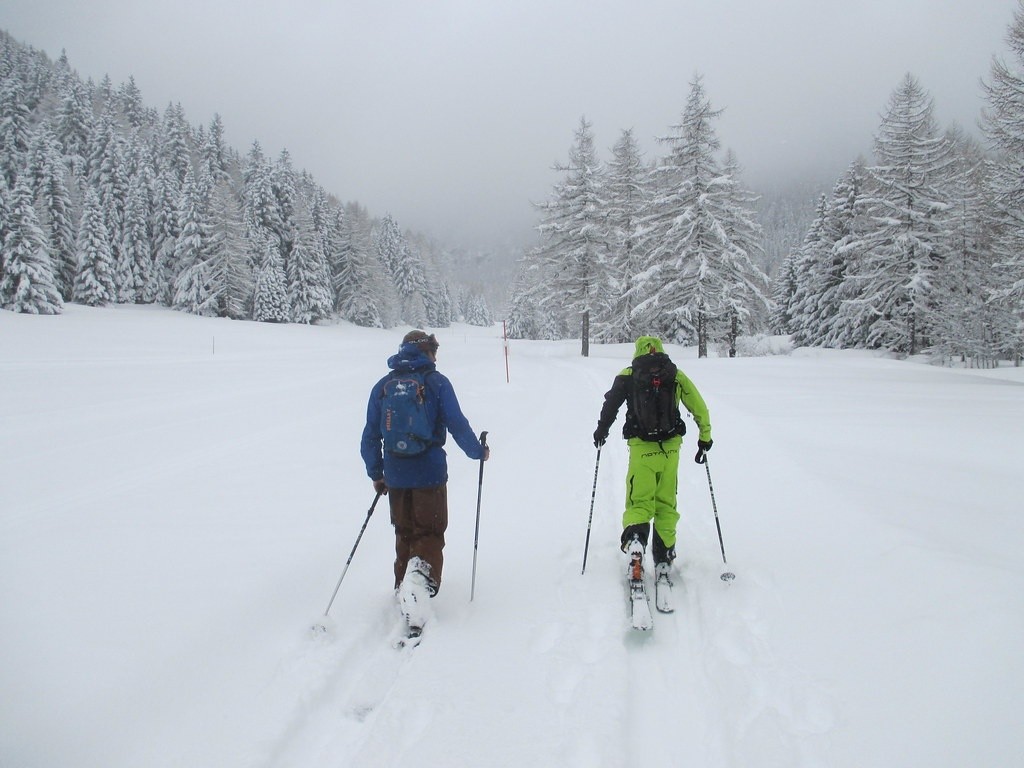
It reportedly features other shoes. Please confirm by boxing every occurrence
[401,593,421,633]
[654,560,671,585]
[627,541,645,583]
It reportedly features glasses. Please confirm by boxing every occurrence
[406,334,440,346]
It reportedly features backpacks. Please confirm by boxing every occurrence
[626,353,680,442]
[382,369,446,458]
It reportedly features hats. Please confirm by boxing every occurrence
[402,330,438,351]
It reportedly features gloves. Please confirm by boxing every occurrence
[695,439,713,464]
[594,420,609,448]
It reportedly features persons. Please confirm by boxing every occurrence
[360,327,489,650]
[593,331,713,639]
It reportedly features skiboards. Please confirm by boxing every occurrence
[628,573,675,632]
